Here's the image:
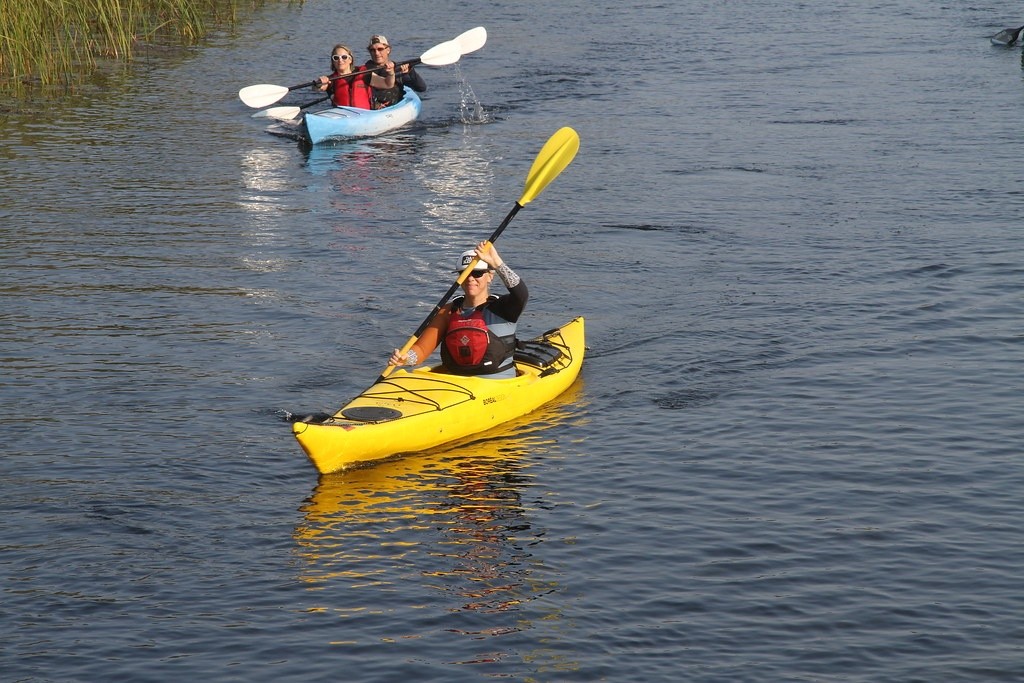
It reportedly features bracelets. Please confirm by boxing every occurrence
[388,74,395,76]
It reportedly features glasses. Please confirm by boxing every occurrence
[331,55,351,62]
[457,269,488,279]
[370,47,387,52]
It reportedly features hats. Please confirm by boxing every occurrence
[367,33,389,47]
[451,249,495,275]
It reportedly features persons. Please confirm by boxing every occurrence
[312,42,396,111]
[364,35,426,110]
[386,240,529,380]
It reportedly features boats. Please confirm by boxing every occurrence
[293,314,585,474]
[303,85,424,145]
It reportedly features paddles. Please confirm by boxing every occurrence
[238,23,488,120]
[238,39,462,110]
[370,123,581,386]
